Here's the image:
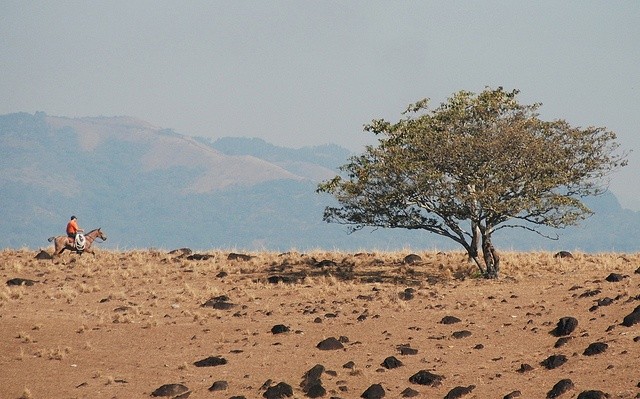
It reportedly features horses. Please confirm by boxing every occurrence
[48,227,107,259]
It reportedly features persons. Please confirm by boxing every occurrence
[67,216,84,239]
